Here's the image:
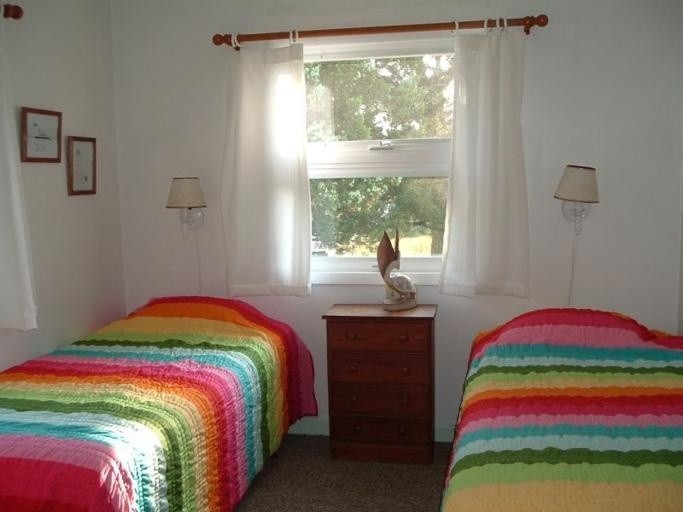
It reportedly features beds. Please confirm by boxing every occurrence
[0,296,317,512]
[440,308,683,512]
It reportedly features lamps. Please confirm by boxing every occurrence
[166,177,207,242]
[553,164,599,237]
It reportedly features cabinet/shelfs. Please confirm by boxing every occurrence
[321,304,437,463]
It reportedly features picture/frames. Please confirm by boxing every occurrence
[67,136,96,195]
[20,107,61,162]
